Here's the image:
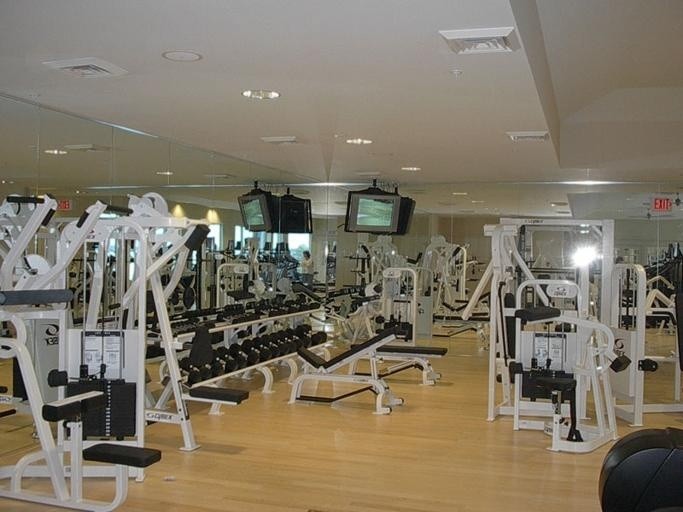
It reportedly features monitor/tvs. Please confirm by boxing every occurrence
[344,187,416,236]
[237,189,312,234]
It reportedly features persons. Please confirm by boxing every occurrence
[302,251,314,288]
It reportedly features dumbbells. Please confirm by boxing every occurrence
[168,293,328,386]
